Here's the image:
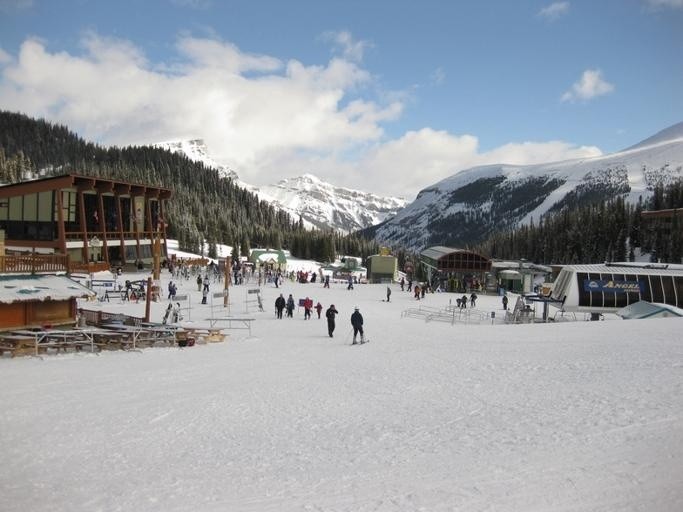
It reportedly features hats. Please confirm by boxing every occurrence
[355,304,360,310]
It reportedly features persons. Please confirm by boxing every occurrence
[349,306,365,344]
[324,303,338,338]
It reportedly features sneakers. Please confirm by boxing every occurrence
[328,334,366,344]
